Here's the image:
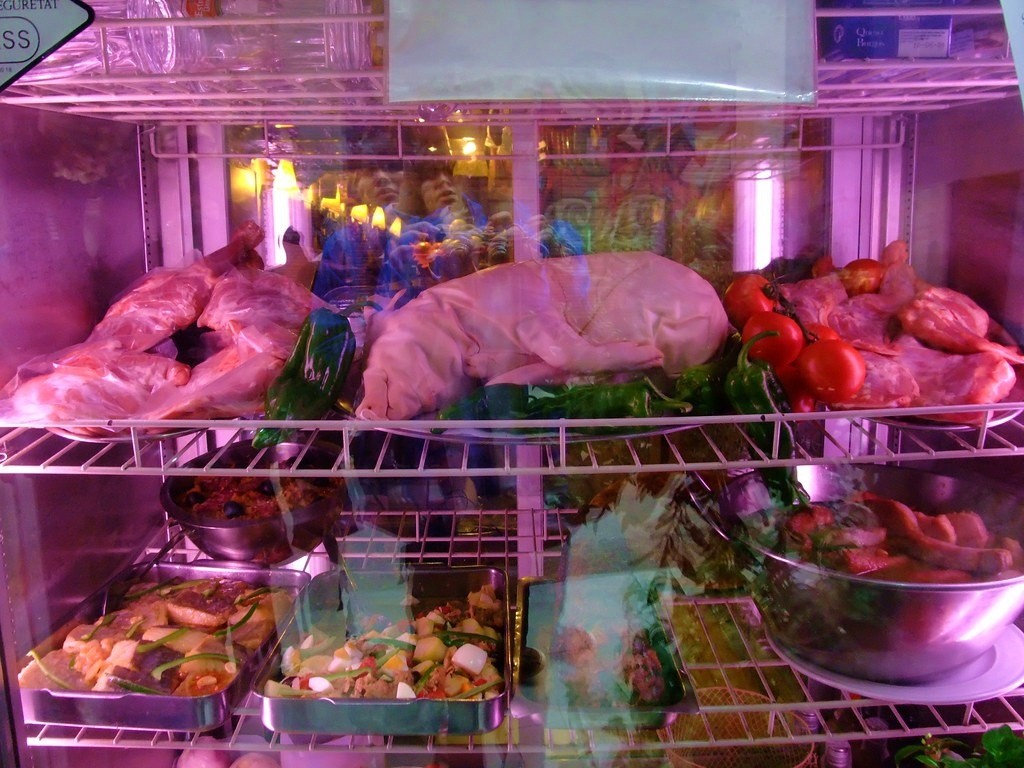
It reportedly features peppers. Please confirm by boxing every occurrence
[249,296,800,485]
[24,572,505,700]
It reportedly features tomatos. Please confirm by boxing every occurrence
[723,272,865,416]
[815,254,886,295]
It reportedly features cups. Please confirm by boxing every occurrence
[9,1,376,116]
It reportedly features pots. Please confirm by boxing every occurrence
[669,463,1024,684]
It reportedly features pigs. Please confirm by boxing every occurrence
[354,250,730,425]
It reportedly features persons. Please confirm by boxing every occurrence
[317,133,567,768]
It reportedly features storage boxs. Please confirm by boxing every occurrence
[814,0,956,65]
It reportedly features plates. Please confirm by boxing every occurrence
[764,621,1024,705]
[868,385,1024,431]
[48,427,204,445]
[344,410,705,446]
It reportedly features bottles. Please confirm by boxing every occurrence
[799,677,918,768]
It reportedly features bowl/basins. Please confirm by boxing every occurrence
[159,442,348,566]
